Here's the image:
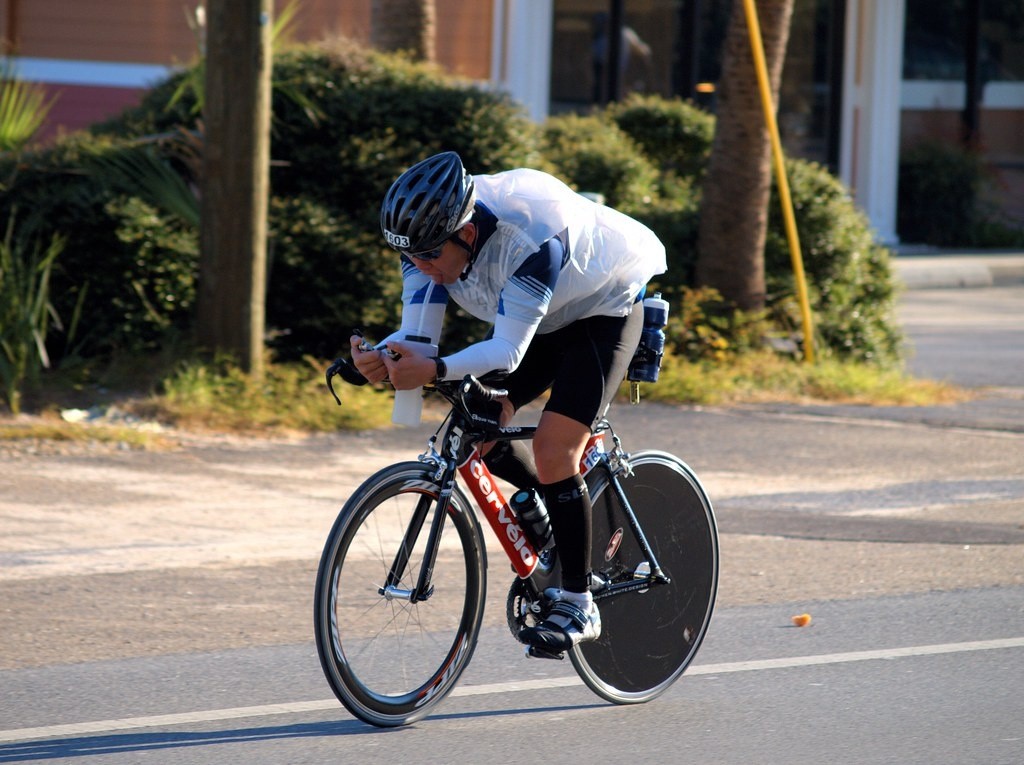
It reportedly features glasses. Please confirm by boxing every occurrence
[412,250,441,261]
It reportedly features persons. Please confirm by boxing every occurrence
[351,152,667,649]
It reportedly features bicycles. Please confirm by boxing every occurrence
[313,327,722,730]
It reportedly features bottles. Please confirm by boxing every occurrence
[510,488,555,550]
[627,292,670,382]
[392,335,432,426]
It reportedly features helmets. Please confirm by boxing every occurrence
[380,152,477,256]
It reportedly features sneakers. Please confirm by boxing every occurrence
[518,590,602,648]
